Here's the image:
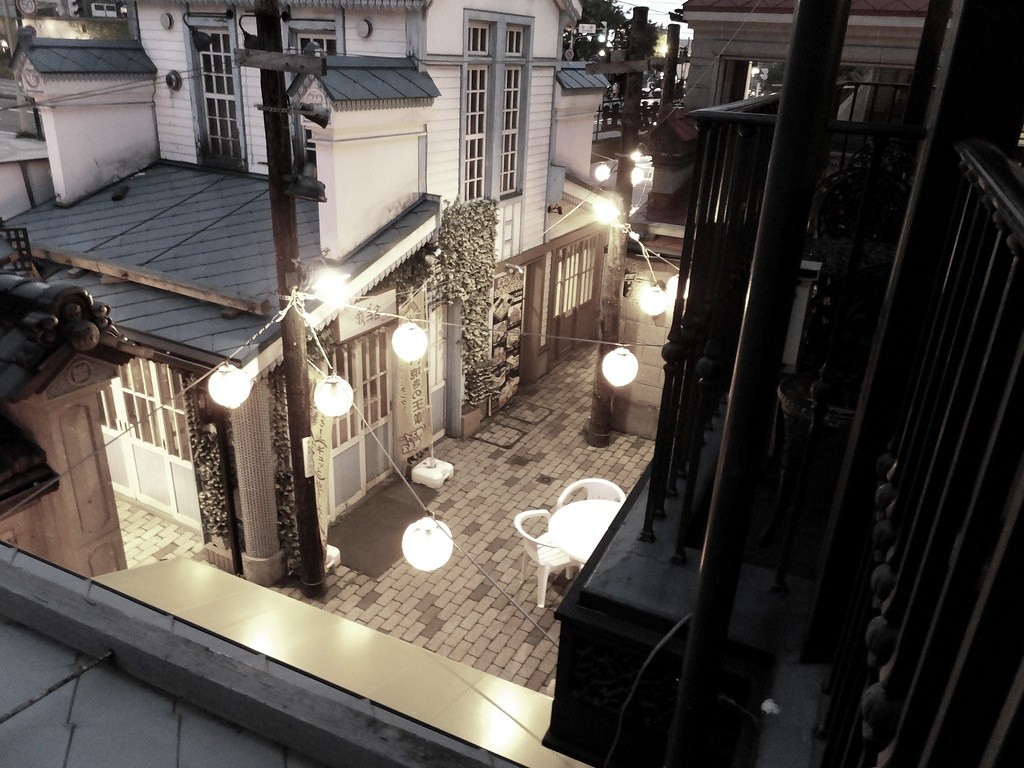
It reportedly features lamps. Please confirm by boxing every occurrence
[183,9,234,52]
[238,10,291,50]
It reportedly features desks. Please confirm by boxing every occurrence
[547,499,622,564]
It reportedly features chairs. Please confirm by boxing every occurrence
[513,509,581,609]
[755,139,914,549]
[555,477,626,510]
